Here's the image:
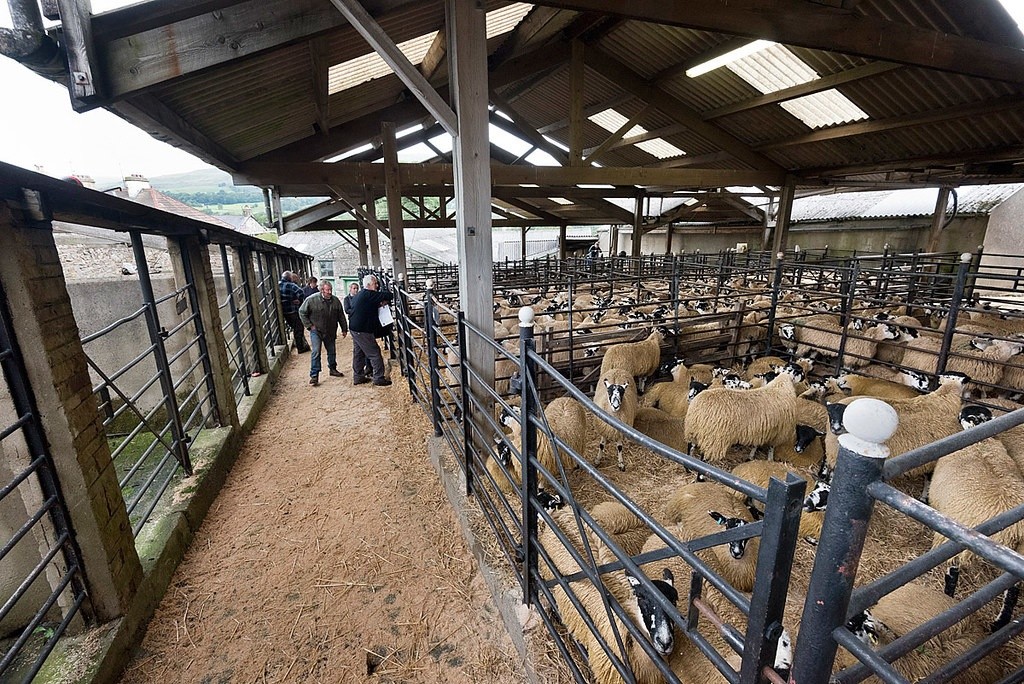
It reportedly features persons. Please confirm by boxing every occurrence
[299,280,349,385]
[278,271,319,354]
[589,241,602,258]
[344,274,393,387]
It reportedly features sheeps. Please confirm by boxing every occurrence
[447,272,1024,684]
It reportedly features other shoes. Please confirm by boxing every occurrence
[374,380,392,386]
[364,368,372,376]
[309,377,318,383]
[330,368,343,377]
[353,377,371,385]
[298,346,310,354]
